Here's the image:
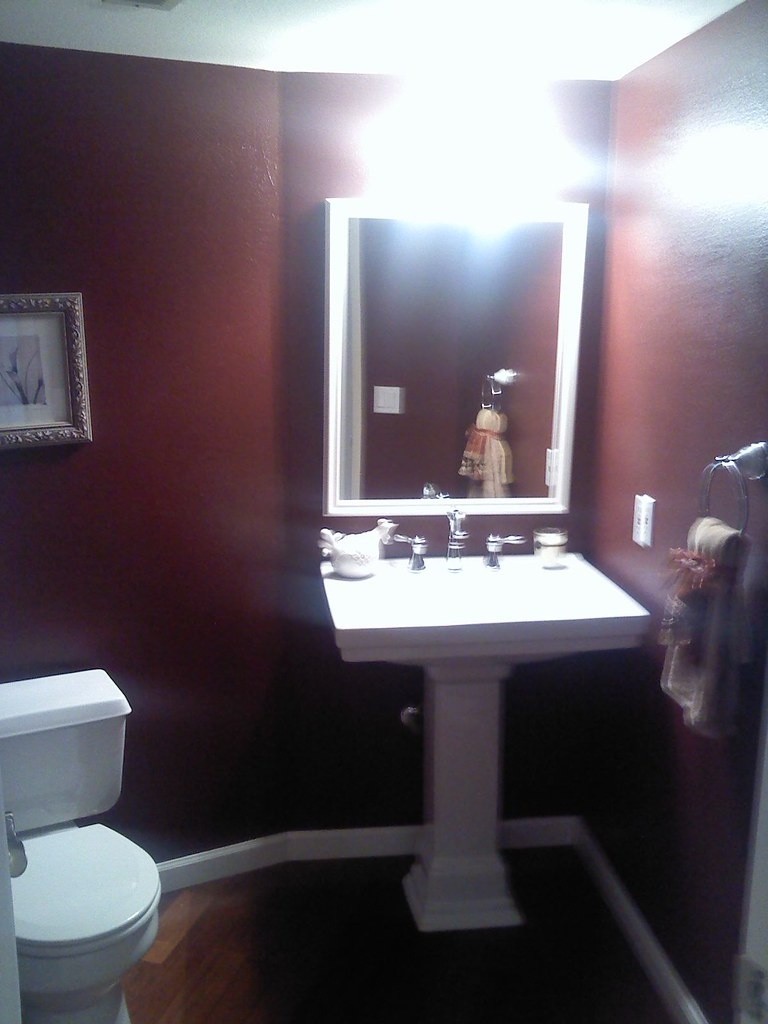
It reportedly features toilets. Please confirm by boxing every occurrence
[0,667,162,1024]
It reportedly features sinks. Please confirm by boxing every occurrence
[317,552,653,662]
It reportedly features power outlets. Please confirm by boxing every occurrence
[632,493,656,548]
[546,449,553,488]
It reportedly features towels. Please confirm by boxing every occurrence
[458,409,514,498]
[658,514,754,746]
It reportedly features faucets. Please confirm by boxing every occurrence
[445,510,471,572]
[422,480,450,499]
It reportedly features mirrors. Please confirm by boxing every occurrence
[324,195,588,516]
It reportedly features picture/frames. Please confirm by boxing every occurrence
[0,293,93,452]
